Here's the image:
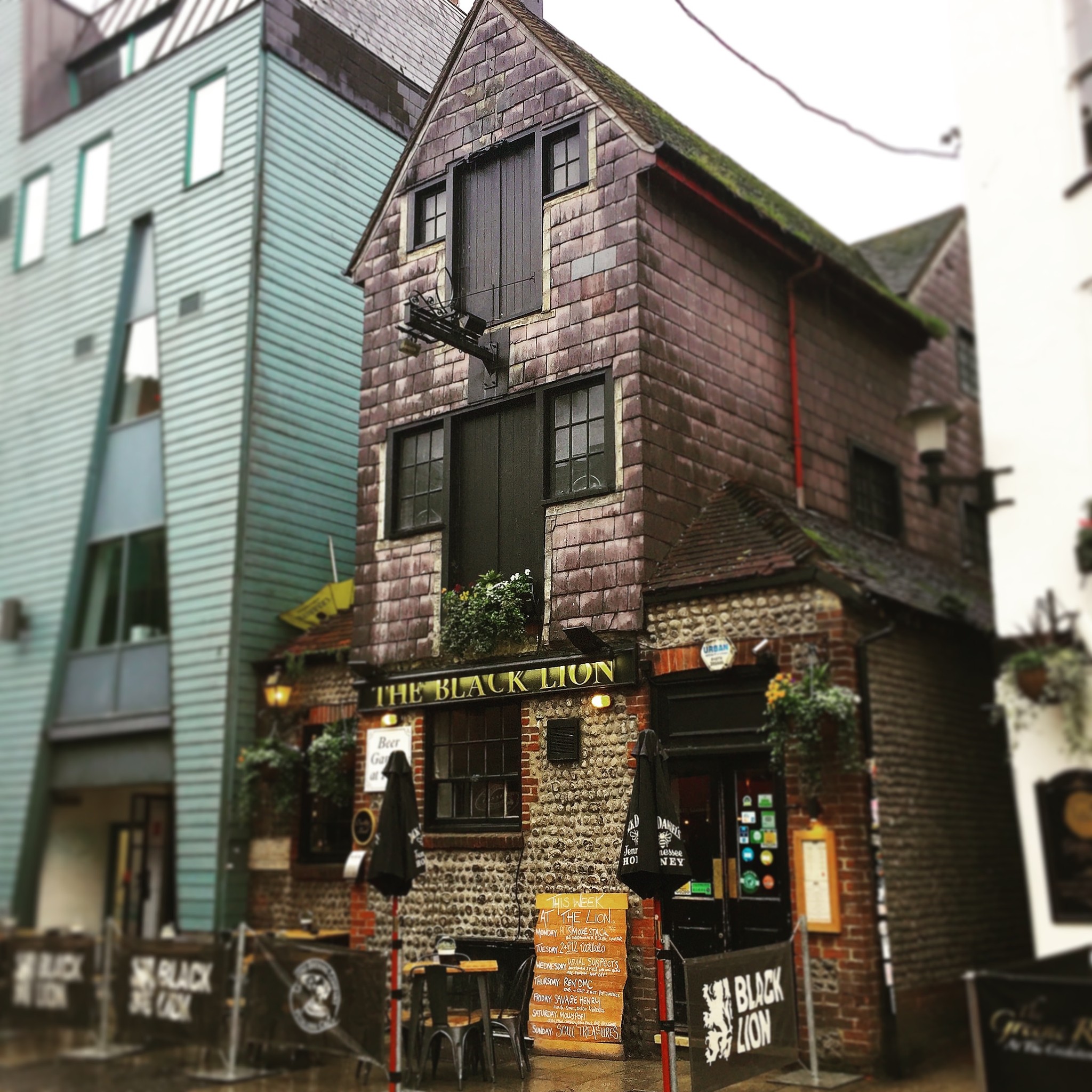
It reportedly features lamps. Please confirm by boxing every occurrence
[261,665,310,732]
[394,267,498,377]
[753,638,779,673]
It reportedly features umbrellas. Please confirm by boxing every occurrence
[616,730,692,1092]
[366,752,426,1092]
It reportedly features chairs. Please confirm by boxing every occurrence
[375,937,535,1092]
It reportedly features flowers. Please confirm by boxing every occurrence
[758,655,870,810]
[440,569,532,653]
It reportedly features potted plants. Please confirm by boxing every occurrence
[1004,634,1090,752]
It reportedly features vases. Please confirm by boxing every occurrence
[788,713,838,752]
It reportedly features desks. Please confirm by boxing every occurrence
[402,957,500,1083]
[230,926,350,1070]
